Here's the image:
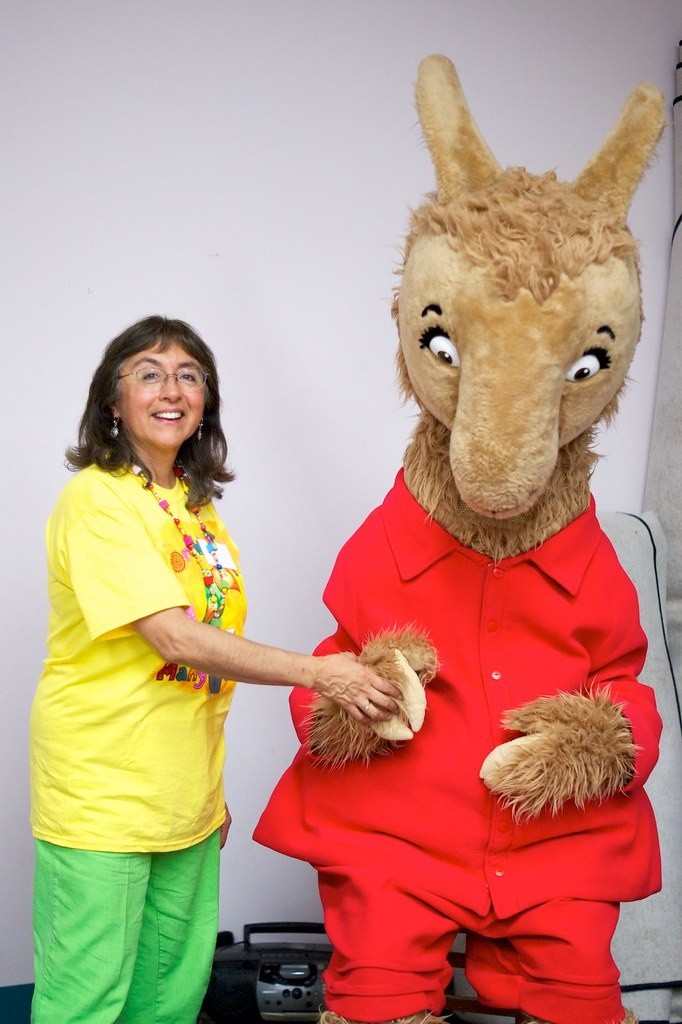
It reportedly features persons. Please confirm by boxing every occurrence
[30,316,404,1024]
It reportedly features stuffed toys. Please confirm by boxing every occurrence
[251,56,664,1024]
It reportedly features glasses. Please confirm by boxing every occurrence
[117,367,210,390]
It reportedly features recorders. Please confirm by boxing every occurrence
[196,921,335,1024]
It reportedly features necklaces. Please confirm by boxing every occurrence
[125,460,229,621]
